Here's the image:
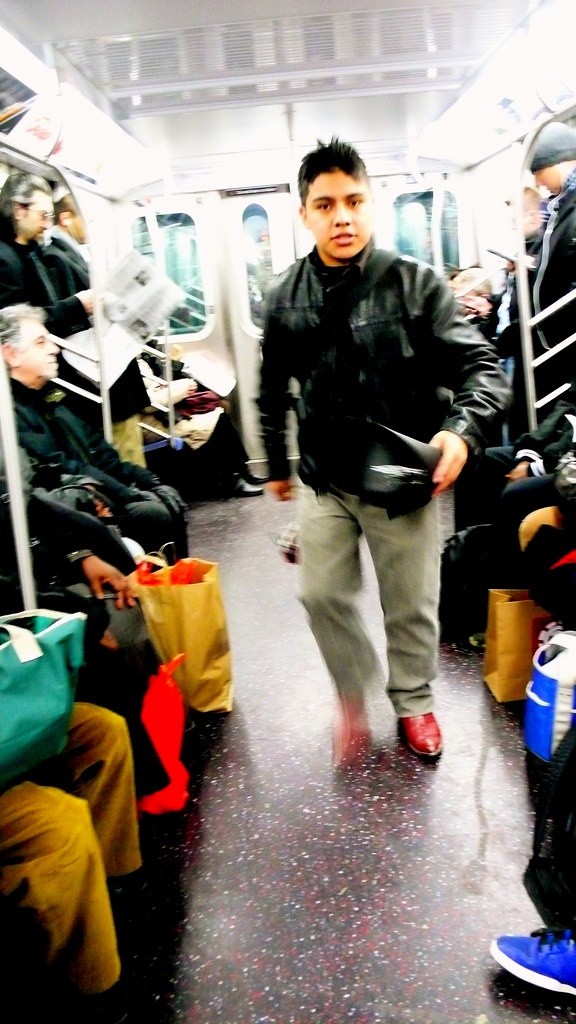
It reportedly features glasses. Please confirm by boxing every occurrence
[24,207,55,222]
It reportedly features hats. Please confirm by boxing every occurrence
[345,411,442,511]
[529,121,576,174]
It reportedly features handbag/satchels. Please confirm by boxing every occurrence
[0,608,87,787]
[125,543,234,714]
[134,654,190,814]
[482,588,550,703]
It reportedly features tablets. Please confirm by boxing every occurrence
[486,248,537,271]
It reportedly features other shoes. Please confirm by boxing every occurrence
[245,472,269,483]
[400,712,442,756]
[233,478,263,496]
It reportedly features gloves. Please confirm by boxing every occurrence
[137,490,163,505]
[147,476,190,513]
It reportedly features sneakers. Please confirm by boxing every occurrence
[489,927,576,996]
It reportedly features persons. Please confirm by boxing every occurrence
[1,171,258,1022]
[258,137,511,772]
[443,117,573,998]
